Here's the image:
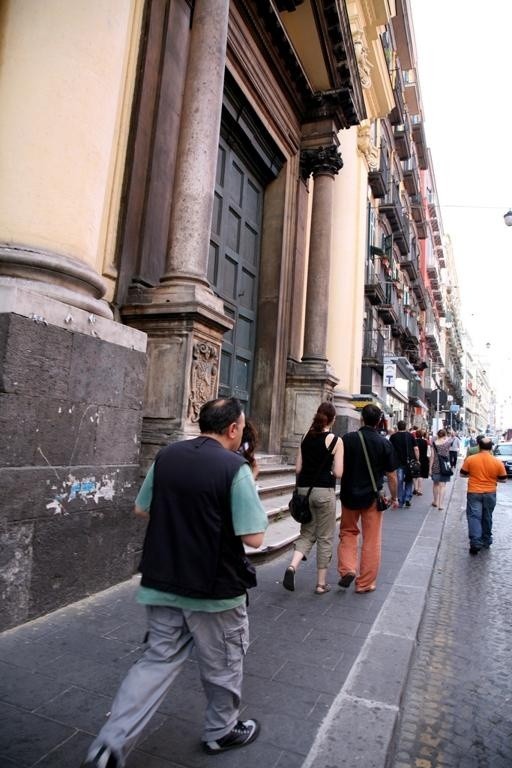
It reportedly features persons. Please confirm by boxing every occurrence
[234,421,258,481]
[466,435,492,455]
[390,421,459,511]
[78,398,270,767]
[283,402,344,594]
[337,403,399,594]
[459,437,508,555]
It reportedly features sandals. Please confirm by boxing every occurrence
[314,583,332,594]
[282,565,295,592]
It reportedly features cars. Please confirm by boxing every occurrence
[492,441,512,475]
[478,428,502,451]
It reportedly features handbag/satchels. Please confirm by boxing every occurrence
[375,487,387,512]
[439,460,453,477]
[288,494,312,525]
[405,460,423,477]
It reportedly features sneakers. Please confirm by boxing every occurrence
[199,715,261,755]
[355,585,377,594]
[397,489,445,511]
[80,744,119,768]
[482,542,490,549]
[469,543,482,555]
[336,569,356,589]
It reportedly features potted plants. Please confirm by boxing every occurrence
[381,255,418,318]
